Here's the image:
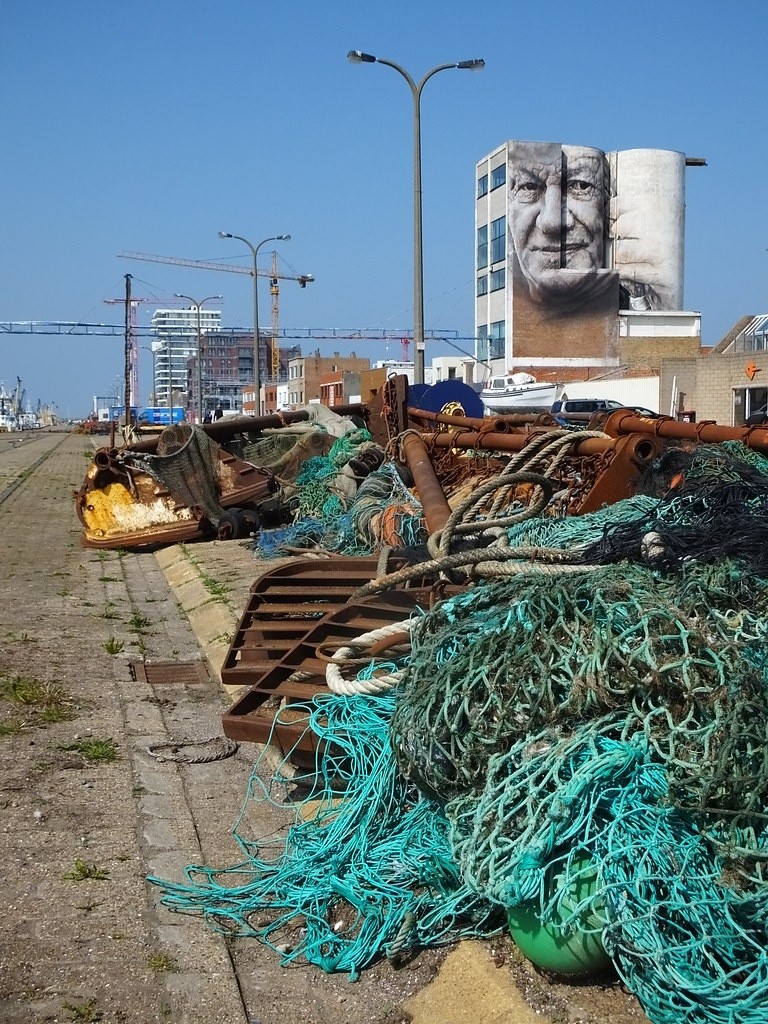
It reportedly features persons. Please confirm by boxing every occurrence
[508,140,686,325]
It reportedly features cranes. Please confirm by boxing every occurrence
[104,295,224,402]
[115,248,315,384]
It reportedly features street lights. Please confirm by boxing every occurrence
[174,292,223,425]
[346,46,488,386]
[217,230,292,417]
[139,344,168,410]
